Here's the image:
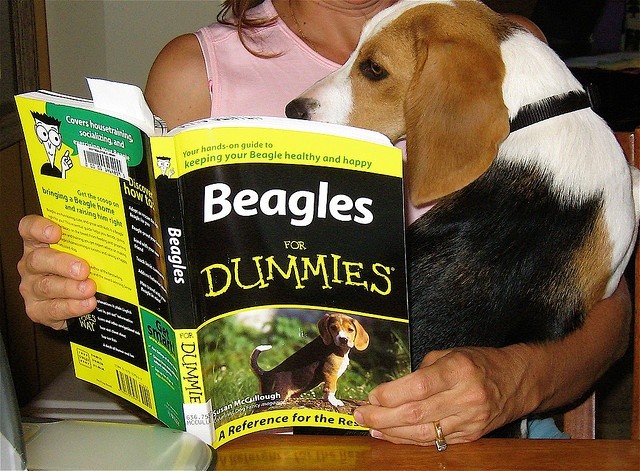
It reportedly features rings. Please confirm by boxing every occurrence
[433,421,448,453]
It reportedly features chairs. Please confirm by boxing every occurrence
[563,127,640,439]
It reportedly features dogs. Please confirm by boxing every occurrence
[250,314,370,413]
[284,1,639,437]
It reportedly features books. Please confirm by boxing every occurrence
[14,76,412,450]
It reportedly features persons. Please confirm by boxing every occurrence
[17,0,634,447]
